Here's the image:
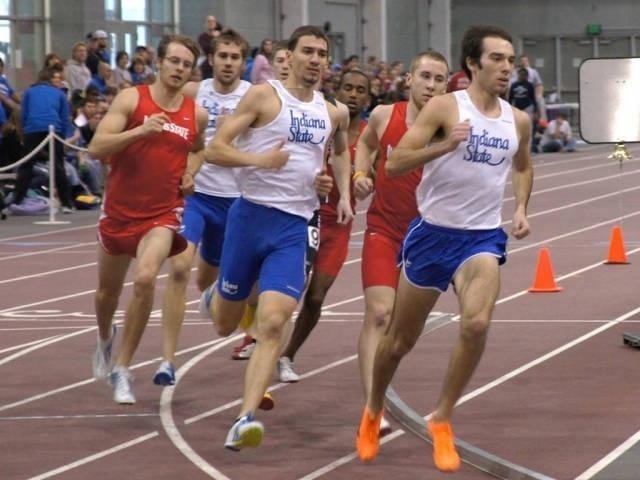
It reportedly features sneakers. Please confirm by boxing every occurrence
[91,325,118,381]
[272,356,302,383]
[258,392,274,411]
[232,335,258,359]
[426,417,462,473]
[354,401,385,464]
[108,366,136,404]
[223,410,264,451]
[199,280,222,316]
[381,418,393,436]
[153,362,177,387]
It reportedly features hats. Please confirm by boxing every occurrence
[87,33,99,39]
[95,30,109,39]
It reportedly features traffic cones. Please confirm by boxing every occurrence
[527,247,563,294]
[602,225,632,265]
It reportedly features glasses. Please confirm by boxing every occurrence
[160,55,196,70]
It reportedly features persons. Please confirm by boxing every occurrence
[88,36,209,407]
[232,41,292,361]
[275,68,369,382]
[1,15,576,219]
[354,50,448,439]
[153,34,252,389]
[355,24,533,471]
[197,26,341,453]
